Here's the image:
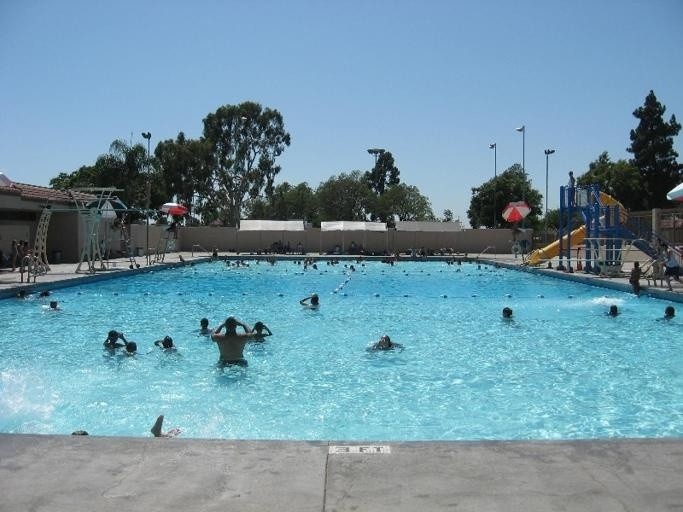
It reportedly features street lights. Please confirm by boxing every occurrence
[516,123,528,229]
[490,142,499,228]
[143,132,153,222]
[544,148,556,245]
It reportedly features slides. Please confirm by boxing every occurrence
[523,189,627,266]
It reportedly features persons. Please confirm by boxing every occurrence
[153,335,176,353]
[367,335,402,350]
[502,307,512,318]
[665,306,675,318]
[71,415,182,437]
[610,305,618,315]
[194,318,214,335]
[563,170,576,207]
[252,321,272,342]
[630,261,642,297]
[10,222,498,300]
[299,293,321,311]
[103,330,137,355]
[49,300,57,308]
[655,237,683,291]
[211,315,254,367]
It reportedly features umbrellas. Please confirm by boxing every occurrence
[161,203,188,214]
[502,201,531,223]
